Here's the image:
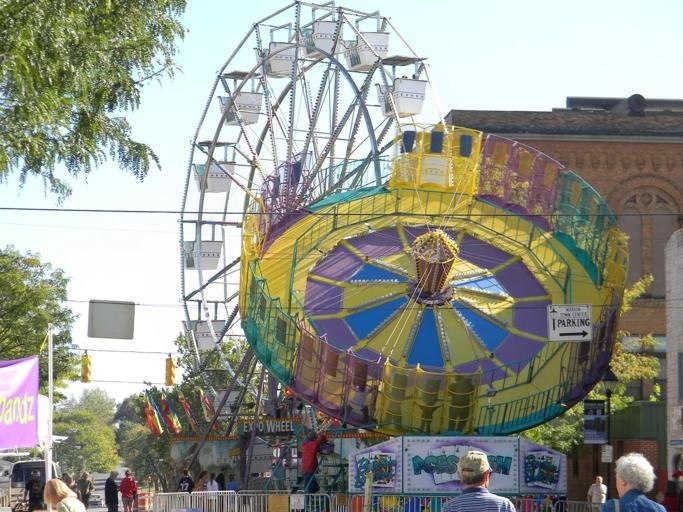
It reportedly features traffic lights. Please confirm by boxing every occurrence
[81,354,91,383]
[165,357,178,387]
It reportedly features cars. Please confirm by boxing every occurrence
[88,493,103,507]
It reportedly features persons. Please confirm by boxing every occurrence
[104,470,121,512]
[380,389,417,431]
[445,374,474,431]
[585,475,606,512]
[600,451,666,512]
[118,470,137,512]
[337,385,377,424]
[22,469,93,511]
[299,426,327,494]
[441,450,516,512]
[410,399,443,435]
[176,468,239,500]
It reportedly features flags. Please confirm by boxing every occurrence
[198,391,219,430]
[160,388,182,433]
[178,390,198,434]
[142,389,166,437]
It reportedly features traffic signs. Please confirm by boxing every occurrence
[547,304,592,343]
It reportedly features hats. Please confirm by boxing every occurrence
[458,450,490,476]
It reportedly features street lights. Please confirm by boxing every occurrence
[601,366,618,499]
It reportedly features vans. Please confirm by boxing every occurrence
[1,460,62,512]
